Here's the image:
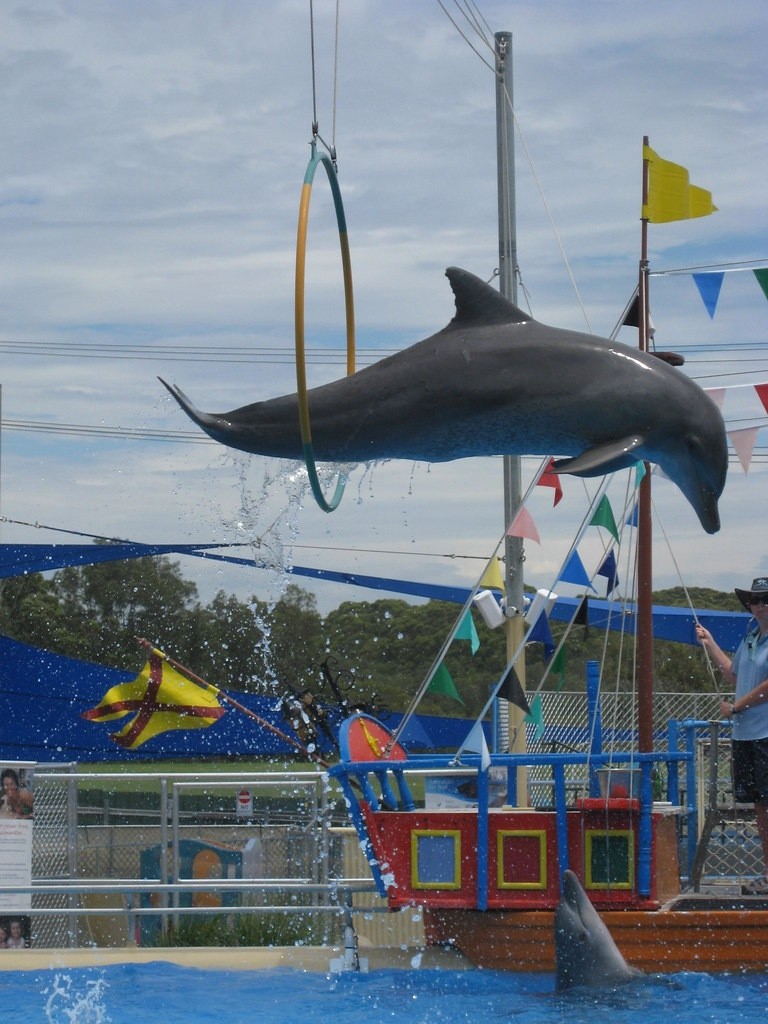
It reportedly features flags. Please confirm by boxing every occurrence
[80,652,226,748]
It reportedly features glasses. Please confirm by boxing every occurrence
[750,595,768,606]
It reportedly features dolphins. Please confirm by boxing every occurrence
[554,870,686,1010]
[157,267,728,534]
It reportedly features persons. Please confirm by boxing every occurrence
[0,926,6,949]
[8,918,27,950]
[1,768,35,820]
[696,577,767,896]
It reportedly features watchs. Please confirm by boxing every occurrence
[729,703,737,714]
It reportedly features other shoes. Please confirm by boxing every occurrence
[741,877,768,895]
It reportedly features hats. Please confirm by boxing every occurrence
[735,577,768,614]
